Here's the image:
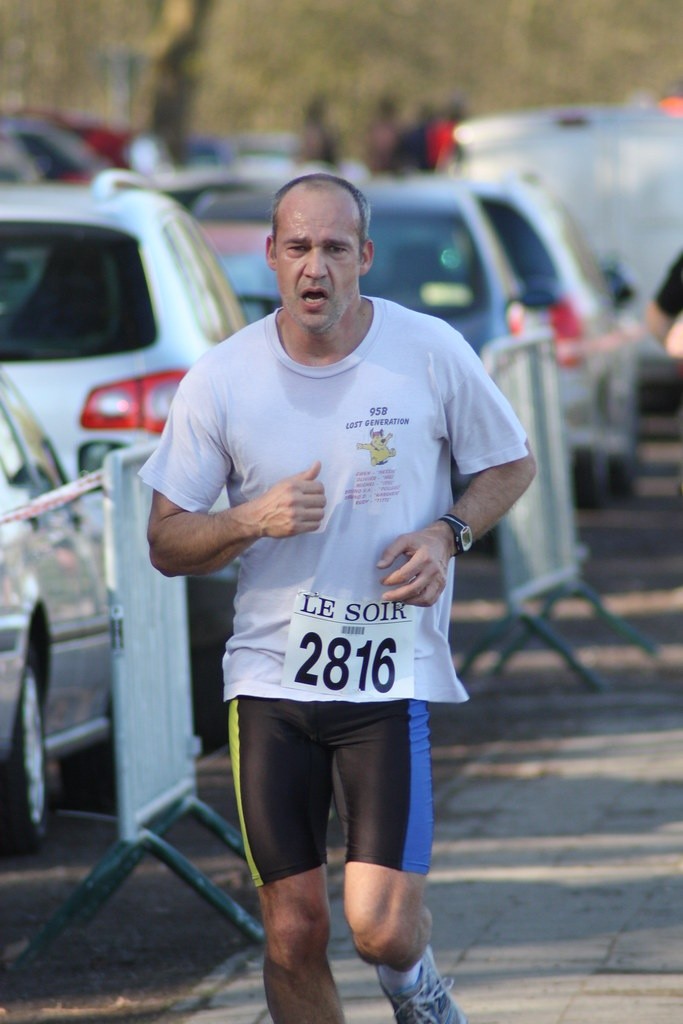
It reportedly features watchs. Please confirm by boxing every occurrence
[438,514,473,557]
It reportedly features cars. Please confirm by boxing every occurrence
[1,367,113,857]
[0,101,683,543]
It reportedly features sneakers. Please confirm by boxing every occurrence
[375,944,468,1024]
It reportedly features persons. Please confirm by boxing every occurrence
[136,177,538,1024]
[646,251,683,357]
[362,99,462,175]
[303,98,335,166]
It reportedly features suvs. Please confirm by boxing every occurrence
[0,170,253,666]
